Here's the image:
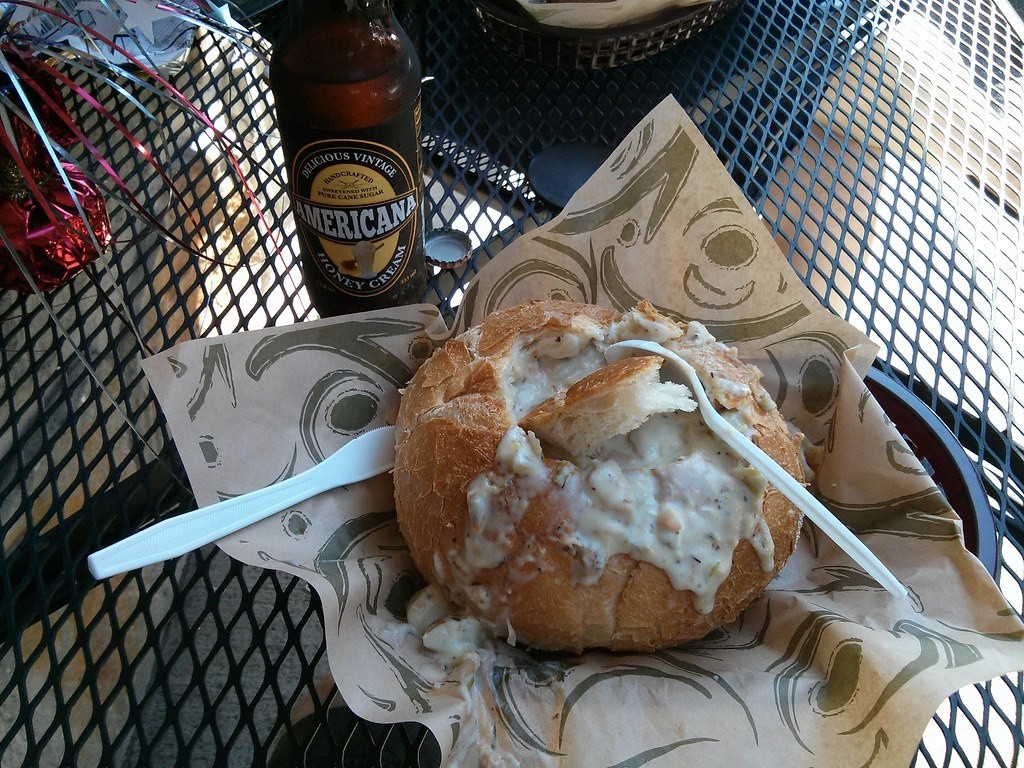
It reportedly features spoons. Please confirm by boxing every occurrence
[604,338,910,602]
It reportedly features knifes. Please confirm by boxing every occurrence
[89,425,397,582]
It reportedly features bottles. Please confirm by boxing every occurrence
[270,0,426,320]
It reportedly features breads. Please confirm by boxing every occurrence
[393,303,807,651]
